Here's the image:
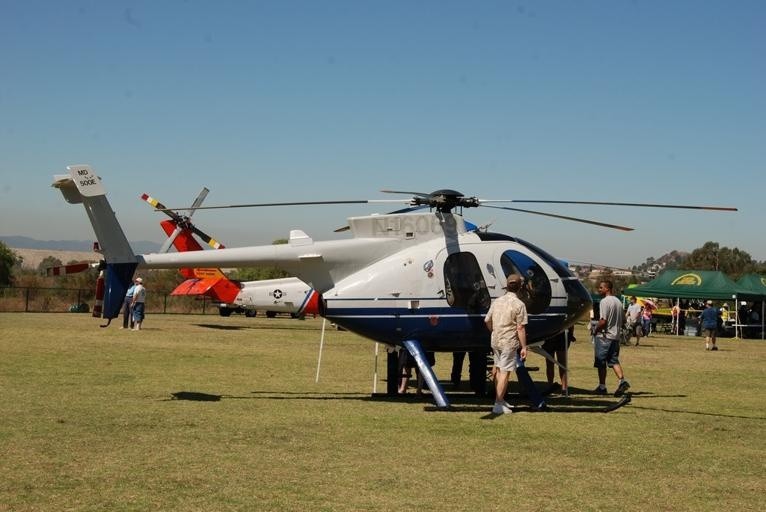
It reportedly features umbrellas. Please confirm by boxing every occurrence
[640,299,657,310]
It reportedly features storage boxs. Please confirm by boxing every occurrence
[683,325,698,337]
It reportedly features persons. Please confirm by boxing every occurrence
[699,300,719,350]
[397,349,435,399]
[544,325,576,396]
[670,301,678,335]
[592,280,630,396]
[641,304,652,338]
[119,282,136,329]
[679,299,702,310]
[720,303,729,326]
[130,277,146,331]
[450,352,466,384]
[752,311,759,336]
[624,295,641,346]
[484,274,528,415]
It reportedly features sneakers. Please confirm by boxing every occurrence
[592,385,607,395]
[493,402,512,415]
[502,400,515,408]
[119,326,141,331]
[614,381,630,397]
[712,346,718,350]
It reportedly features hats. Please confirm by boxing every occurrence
[507,274,522,289]
[136,278,142,283]
